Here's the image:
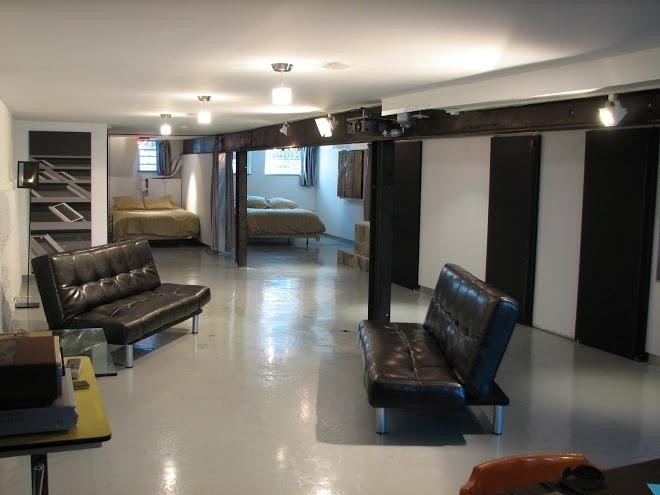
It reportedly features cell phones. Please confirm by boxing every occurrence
[74,380,89,390]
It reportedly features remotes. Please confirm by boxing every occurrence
[66,357,82,380]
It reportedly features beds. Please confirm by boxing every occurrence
[247,174,325,246]
[109,194,200,244]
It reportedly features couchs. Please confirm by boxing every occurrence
[358,262,520,436]
[31,237,210,369]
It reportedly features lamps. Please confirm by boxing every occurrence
[313,113,339,138]
[196,94,213,125]
[270,62,294,110]
[159,113,174,137]
[600,92,627,128]
[15,160,41,308]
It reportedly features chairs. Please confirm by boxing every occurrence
[457,453,598,494]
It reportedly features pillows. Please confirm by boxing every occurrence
[147,194,177,209]
[113,195,144,210]
[270,198,298,208]
[247,196,269,208]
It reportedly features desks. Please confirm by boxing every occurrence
[484,456,660,495]
[51,328,119,378]
[0,355,112,495]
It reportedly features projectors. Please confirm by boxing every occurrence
[346,116,399,134]
